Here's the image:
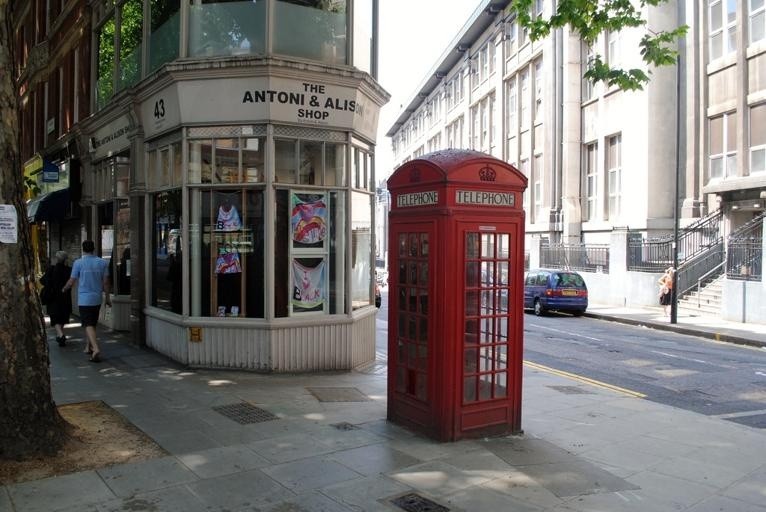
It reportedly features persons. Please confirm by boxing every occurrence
[64,241,113,360]
[38,250,72,348]
[657,267,672,315]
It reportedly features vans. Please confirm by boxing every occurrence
[523,269,588,317]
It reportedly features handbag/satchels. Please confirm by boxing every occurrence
[40,286,47,305]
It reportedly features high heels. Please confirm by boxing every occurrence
[56,335,65,347]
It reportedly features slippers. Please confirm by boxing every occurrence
[82,347,101,361]
